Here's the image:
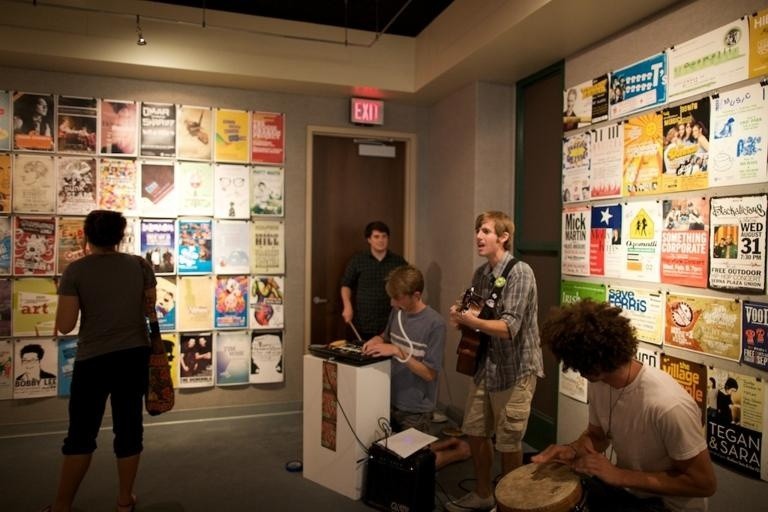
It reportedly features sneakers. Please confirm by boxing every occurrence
[445,492,496,512]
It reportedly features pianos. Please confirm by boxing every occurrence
[308,342,392,366]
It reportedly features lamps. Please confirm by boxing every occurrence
[135,14,147,45]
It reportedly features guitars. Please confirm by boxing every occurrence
[456,286,483,377]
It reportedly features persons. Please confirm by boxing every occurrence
[195,336,212,375]
[713,233,739,258]
[252,182,270,213]
[529,297,719,512]
[610,75,626,108]
[562,89,583,130]
[663,201,706,230]
[360,263,447,440]
[708,375,738,423]
[339,218,410,343]
[20,96,52,141]
[661,117,710,177]
[180,338,199,376]
[611,227,622,244]
[14,343,57,380]
[51,209,165,511]
[145,246,173,272]
[449,212,543,509]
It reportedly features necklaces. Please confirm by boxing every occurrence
[604,357,633,441]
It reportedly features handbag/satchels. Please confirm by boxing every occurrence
[146,354,175,415]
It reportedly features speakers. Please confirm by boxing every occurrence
[363,433,435,512]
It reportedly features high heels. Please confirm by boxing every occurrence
[117,495,135,512]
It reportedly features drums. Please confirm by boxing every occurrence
[494,457,591,512]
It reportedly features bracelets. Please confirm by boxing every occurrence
[561,441,581,461]
